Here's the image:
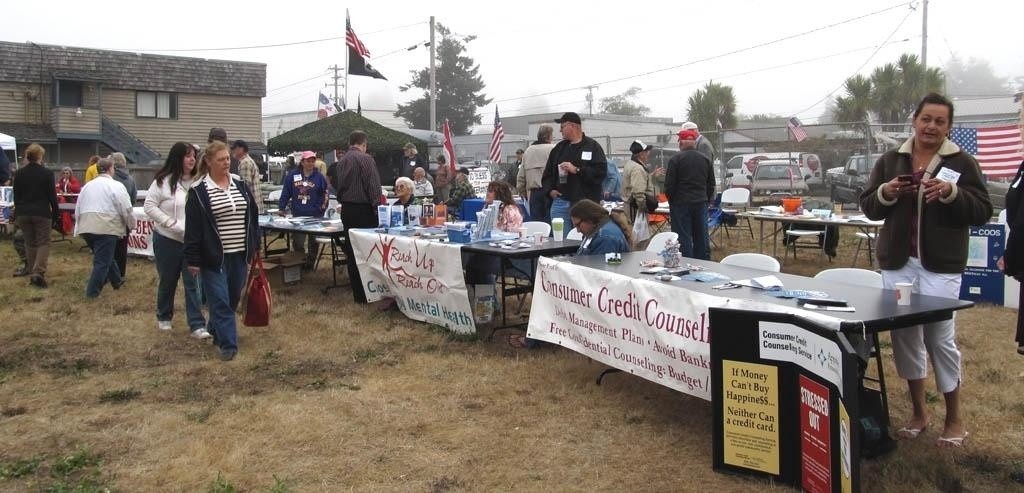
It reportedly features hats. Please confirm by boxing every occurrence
[675,122,698,141]
[515,149,524,155]
[300,151,317,162]
[403,143,417,151]
[630,139,654,154]
[208,127,227,144]
[230,141,249,150]
[555,112,582,124]
[435,154,445,163]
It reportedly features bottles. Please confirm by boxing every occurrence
[834,201,842,215]
[470,223,479,240]
[550,217,565,243]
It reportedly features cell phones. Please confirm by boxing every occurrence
[898,175,912,183]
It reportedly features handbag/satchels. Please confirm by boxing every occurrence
[629,191,659,211]
[236,253,272,327]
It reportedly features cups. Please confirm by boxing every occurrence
[895,284,913,305]
[518,228,527,242]
[558,164,568,185]
[533,232,543,246]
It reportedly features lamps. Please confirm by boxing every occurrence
[73,107,82,117]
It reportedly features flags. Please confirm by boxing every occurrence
[948,124,1024,177]
[489,108,504,164]
[320,92,365,114]
[442,116,456,178]
[345,12,374,56]
[789,115,808,144]
[348,44,384,79]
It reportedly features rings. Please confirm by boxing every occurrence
[936,188,943,197]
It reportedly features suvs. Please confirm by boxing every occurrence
[633,141,828,208]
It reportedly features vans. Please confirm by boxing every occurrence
[265,152,288,171]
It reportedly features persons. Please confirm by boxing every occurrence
[0,144,58,287]
[281,151,328,274]
[603,121,715,258]
[473,181,521,316]
[144,128,261,359]
[996,95,1023,357]
[393,143,475,219]
[330,131,382,305]
[74,152,135,298]
[513,112,608,239]
[55,167,80,201]
[508,200,627,349]
[861,91,996,449]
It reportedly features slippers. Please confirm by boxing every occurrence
[934,428,970,450]
[508,333,527,348]
[895,419,929,441]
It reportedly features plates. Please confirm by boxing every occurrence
[420,232,448,239]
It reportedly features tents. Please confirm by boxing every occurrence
[1,133,18,171]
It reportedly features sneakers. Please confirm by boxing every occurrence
[113,277,128,291]
[158,320,173,331]
[189,325,218,339]
[305,263,314,273]
[354,293,368,304]
[214,343,236,362]
[12,260,32,277]
[30,272,49,290]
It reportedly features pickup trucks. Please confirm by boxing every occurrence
[823,148,885,208]
